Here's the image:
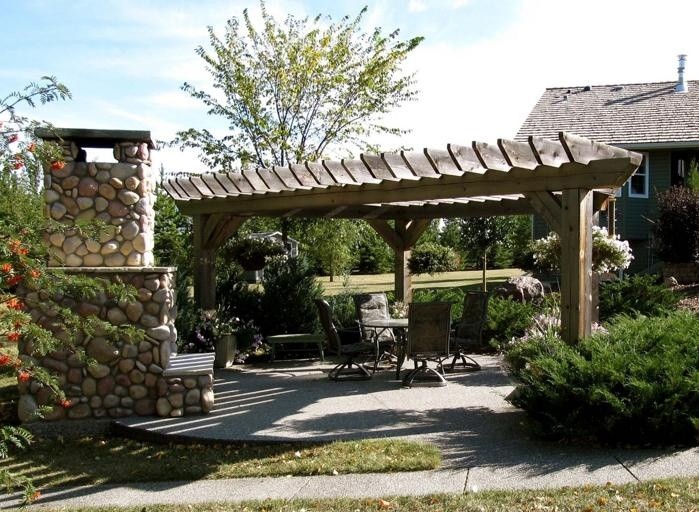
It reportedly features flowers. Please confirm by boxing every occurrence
[186,316,264,364]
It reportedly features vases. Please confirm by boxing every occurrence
[213,335,237,368]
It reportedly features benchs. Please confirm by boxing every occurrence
[266,334,326,362]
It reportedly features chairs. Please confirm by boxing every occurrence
[314,290,490,387]
